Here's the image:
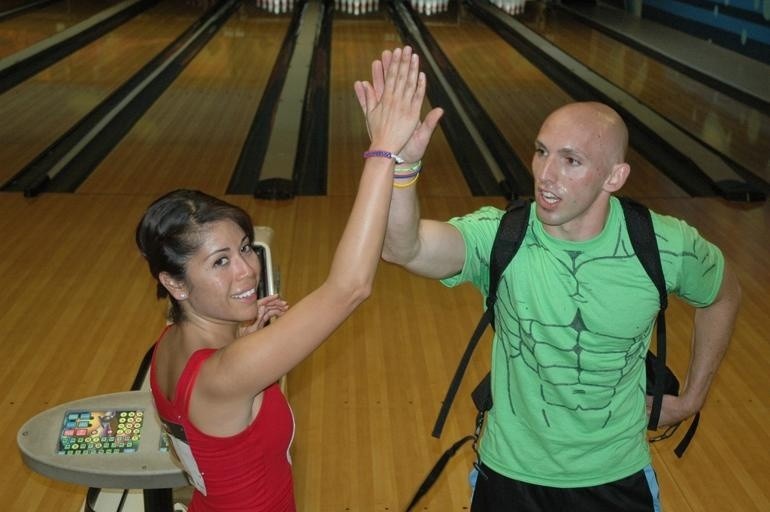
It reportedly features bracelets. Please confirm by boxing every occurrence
[362,151,405,165]
[393,159,422,188]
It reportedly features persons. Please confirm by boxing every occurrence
[355,49,741,512]
[134,45,426,512]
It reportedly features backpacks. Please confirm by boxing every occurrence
[470,198,680,413]
